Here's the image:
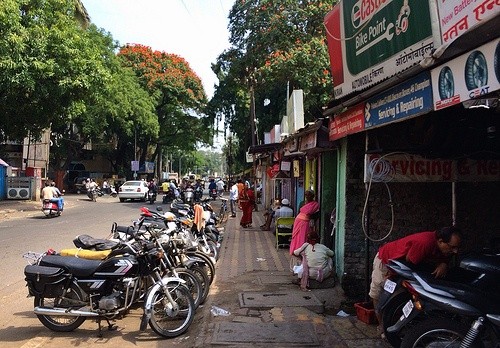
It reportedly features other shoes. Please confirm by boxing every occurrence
[242,224,247,228]
[292,278,302,285]
[248,225,252,227]
[260,224,266,228]
[262,227,270,231]
[230,215,236,217]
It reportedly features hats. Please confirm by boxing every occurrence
[282,199,291,206]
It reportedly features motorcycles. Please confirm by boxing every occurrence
[22,201,221,338]
[375,253,500,348]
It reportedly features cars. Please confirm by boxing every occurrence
[118,180,149,201]
[156,179,170,195]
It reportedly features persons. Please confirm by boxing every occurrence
[161,178,205,199]
[148,178,158,197]
[229,179,244,217]
[260,199,294,248]
[292,231,335,292]
[42,182,63,215]
[228,177,234,192]
[369,226,463,339]
[289,189,320,255]
[208,179,218,197]
[239,180,255,228]
[216,178,224,197]
[86,178,109,195]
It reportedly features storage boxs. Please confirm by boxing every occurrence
[24,264,65,298]
[354,300,377,324]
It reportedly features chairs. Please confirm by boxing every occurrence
[275,216,296,250]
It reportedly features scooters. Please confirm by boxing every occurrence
[148,188,157,204]
[163,184,205,204]
[209,186,218,200]
[41,188,65,218]
[74,184,117,202]
